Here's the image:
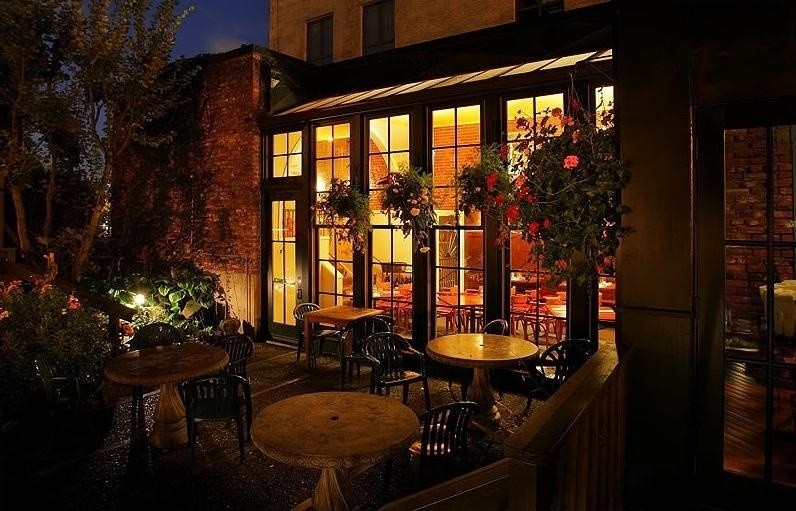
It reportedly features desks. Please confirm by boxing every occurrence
[544,303,616,346]
[759,280,796,343]
[250,390,422,511]
[426,332,537,431]
[104,342,229,448]
[370,288,402,307]
[437,293,485,334]
[302,304,383,376]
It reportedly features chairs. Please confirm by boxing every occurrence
[190,335,256,427]
[723,296,764,361]
[293,303,340,362]
[181,373,252,463]
[522,299,562,348]
[437,296,456,334]
[376,315,395,333]
[527,338,593,409]
[362,333,431,412]
[449,319,510,400]
[461,302,483,332]
[395,401,480,495]
[508,294,528,336]
[337,315,390,392]
[396,293,411,330]
[130,321,183,415]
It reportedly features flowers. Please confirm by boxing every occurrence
[69,309,109,403]
[0,251,80,402]
[453,143,513,216]
[309,176,372,255]
[483,80,635,287]
[375,161,438,255]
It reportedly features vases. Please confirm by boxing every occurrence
[76,403,111,436]
[45,400,74,437]
